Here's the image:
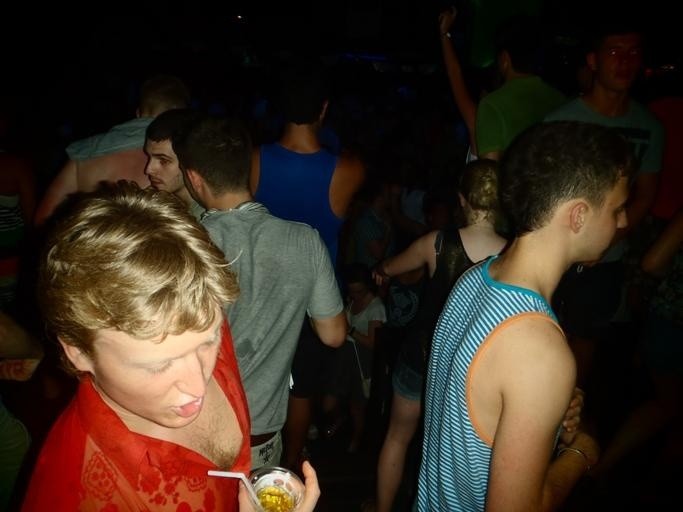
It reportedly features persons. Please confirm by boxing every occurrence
[0,11,683,512]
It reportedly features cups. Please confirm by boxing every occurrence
[245,468,306,512]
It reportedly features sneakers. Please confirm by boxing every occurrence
[323,415,346,439]
